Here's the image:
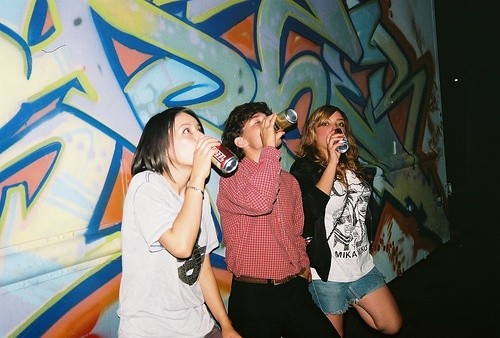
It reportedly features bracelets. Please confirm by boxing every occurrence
[185,186,204,199]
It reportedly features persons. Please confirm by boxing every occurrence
[116,108,239,338]
[217,103,341,338]
[289,106,403,338]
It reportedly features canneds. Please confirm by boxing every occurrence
[331,128,349,153]
[211,140,239,173]
[274,108,298,132]
